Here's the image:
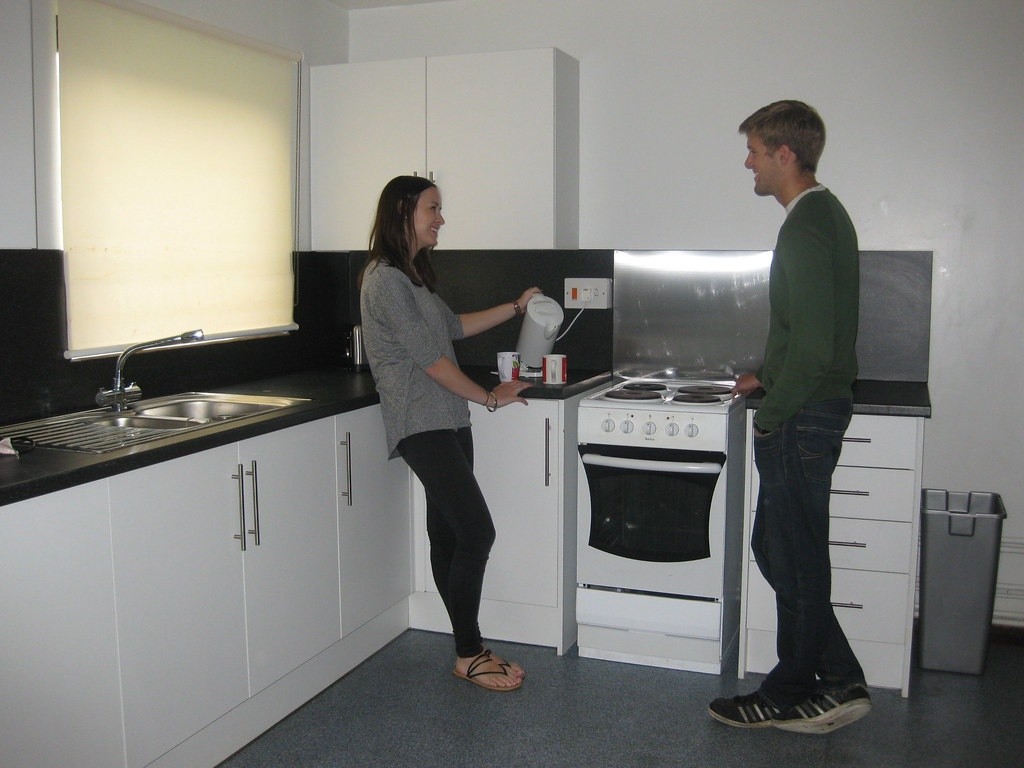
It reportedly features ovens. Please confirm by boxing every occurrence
[575,412,745,674]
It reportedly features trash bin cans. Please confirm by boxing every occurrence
[920,487,1007,675]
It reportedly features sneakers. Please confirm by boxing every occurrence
[708,683,872,734]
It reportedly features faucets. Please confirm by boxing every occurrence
[113,328,205,414]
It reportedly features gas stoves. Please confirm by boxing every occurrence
[578,373,745,409]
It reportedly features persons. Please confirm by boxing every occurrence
[360,175,544,691]
[708,100,872,735]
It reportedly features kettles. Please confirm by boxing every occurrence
[490,296,584,378]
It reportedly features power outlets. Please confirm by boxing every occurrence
[564,277,612,309]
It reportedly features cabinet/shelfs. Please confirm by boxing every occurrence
[737,410,924,699]
[407,382,613,657]
[0,405,407,768]
[309,47,578,249]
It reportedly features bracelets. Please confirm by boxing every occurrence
[511,300,521,316]
[482,391,489,406]
[486,391,497,412]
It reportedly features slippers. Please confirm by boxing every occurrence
[454,647,525,691]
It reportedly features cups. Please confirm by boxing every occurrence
[497,352,521,383]
[543,354,567,384]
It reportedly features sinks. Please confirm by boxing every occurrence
[138,397,311,420]
[91,416,227,431]
